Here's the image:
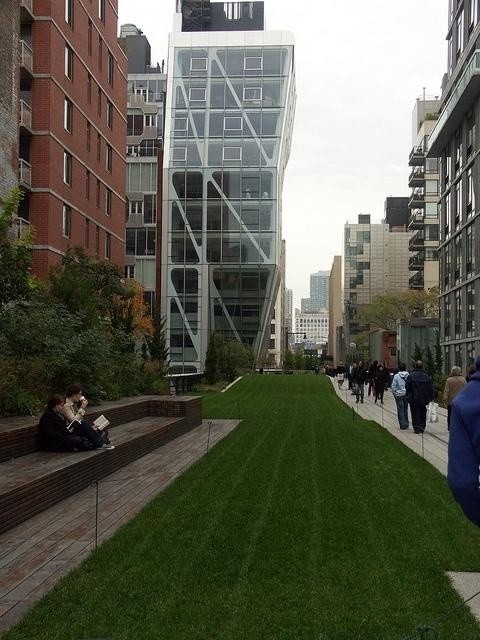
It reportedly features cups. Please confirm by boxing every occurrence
[80,396,88,404]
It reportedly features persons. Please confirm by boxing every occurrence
[38,385,115,452]
[392,360,434,434]
[443,355,480,529]
[336,360,392,404]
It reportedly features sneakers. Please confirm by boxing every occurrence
[351,391,385,404]
[97,443,115,450]
[413,424,423,434]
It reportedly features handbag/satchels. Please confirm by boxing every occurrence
[426,400,438,424]
